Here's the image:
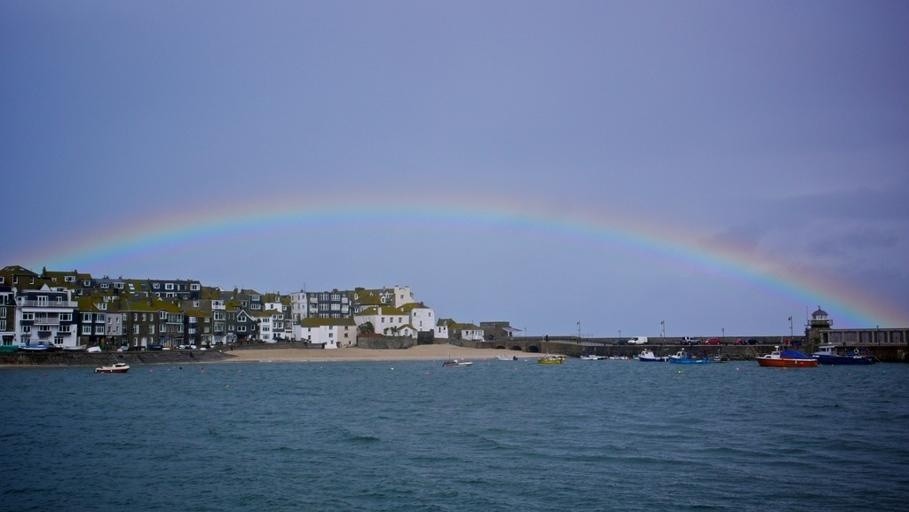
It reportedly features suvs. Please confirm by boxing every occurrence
[679,335,701,345]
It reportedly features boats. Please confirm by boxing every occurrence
[536,354,568,365]
[93,361,131,374]
[442,349,473,368]
[812,342,880,365]
[496,354,519,361]
[578,349,730,364]
[752,345,819,368]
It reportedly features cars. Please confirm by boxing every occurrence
[703,338,720,345]
[23,342,64,352]
[746,338,760,345]
[85,342,218,353]
[733,338,747,345]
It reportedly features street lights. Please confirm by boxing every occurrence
[660,319,666,338]
[576,319,582,337]
[720,326,725,337]
[618,328,622,337]
[787,310,794,336]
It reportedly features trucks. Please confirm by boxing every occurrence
[627,336,649,345]
